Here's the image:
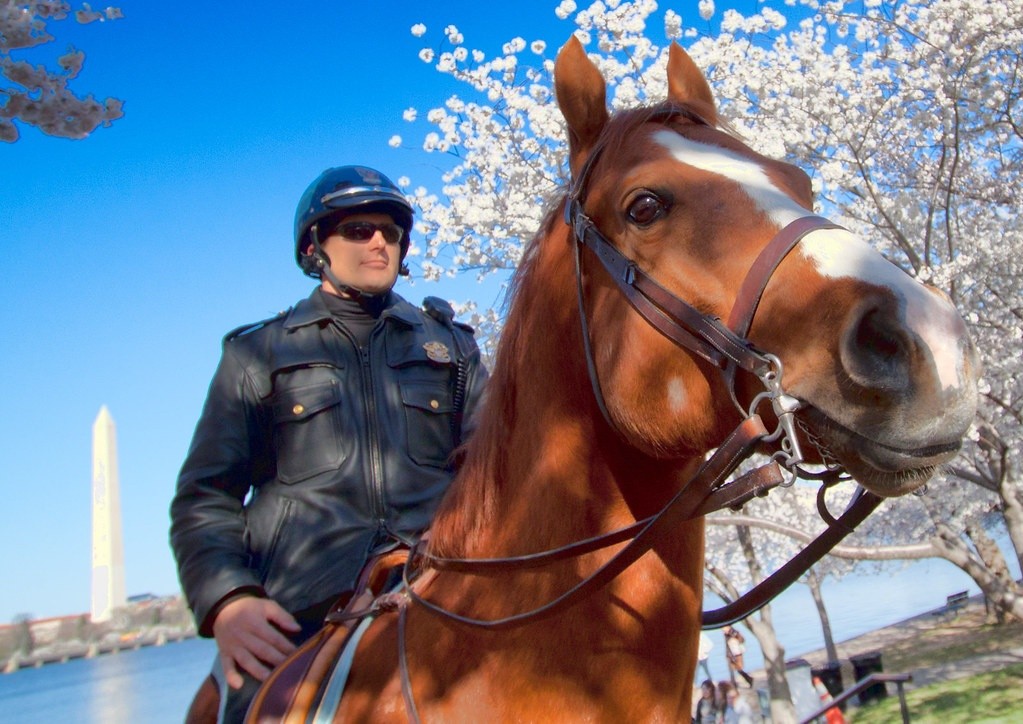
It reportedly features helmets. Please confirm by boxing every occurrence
[293,164,415,278]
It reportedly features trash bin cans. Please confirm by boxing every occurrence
[810,663,846,713]
[849,651,888,705]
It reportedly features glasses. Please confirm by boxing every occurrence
[332,221,404,243]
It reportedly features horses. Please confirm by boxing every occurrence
[182,31,980,724]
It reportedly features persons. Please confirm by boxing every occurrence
[696,680,718,724]
[168,164,491,724]
[698,632,713,679]
[713,682,756,723]
[722,625,753,688]
